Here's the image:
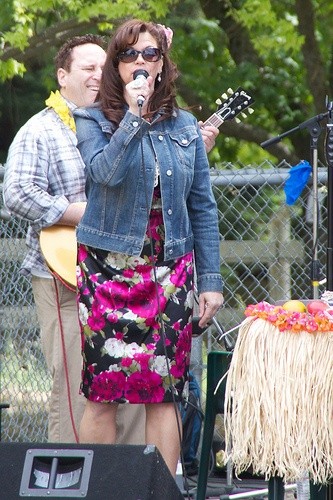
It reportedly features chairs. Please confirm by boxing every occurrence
[196,350,285,500]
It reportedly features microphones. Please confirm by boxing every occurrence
[133,69,149,106]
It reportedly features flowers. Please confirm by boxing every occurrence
[158,23,174,48]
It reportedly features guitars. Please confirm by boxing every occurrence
[38,85,257,294]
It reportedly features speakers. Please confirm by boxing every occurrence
[0,442,185,500]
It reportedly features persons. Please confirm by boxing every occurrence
[177,371,203,477]
[73,19,224,479]
[2,34,220,444]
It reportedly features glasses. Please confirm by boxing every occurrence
[116,46,166,64]
